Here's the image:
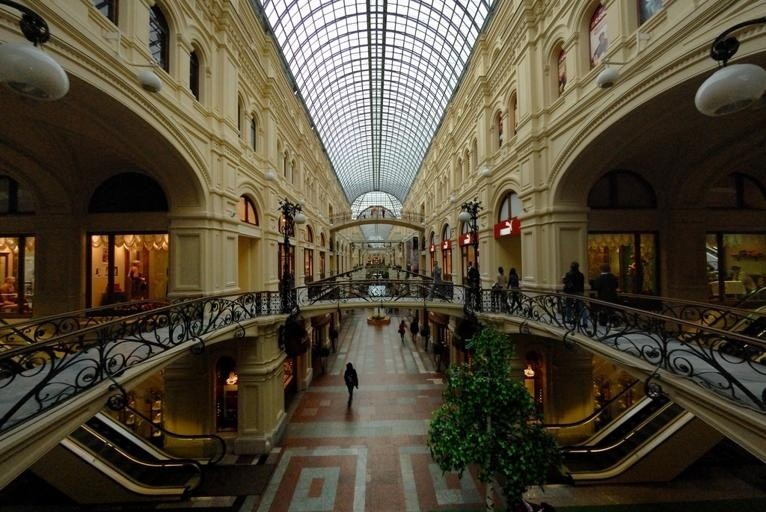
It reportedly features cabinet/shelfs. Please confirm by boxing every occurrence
[104,291,126,305]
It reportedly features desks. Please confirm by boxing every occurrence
[0,294,30,303]
[711,281,747,302]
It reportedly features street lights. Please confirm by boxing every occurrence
[277,198,306,312]
[458,200,483,311]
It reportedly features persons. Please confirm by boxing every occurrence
[490,266,510,311]
[342,362,359,409]
[426,260,446,302]
[396,320,407,343]
[506,267,522,311]
[560,261,585,326]
[408,318,419,343]
[0,274,18,312]
[588,262,622,331]
[126,257,143,295]
[465,260,481,301]
[588,31,608,63]
[731,264,758,293]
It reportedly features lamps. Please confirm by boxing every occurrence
[595,31,648,89]
[105,29,165,94]
[695,16,766,123]
[0,1,70,102]
